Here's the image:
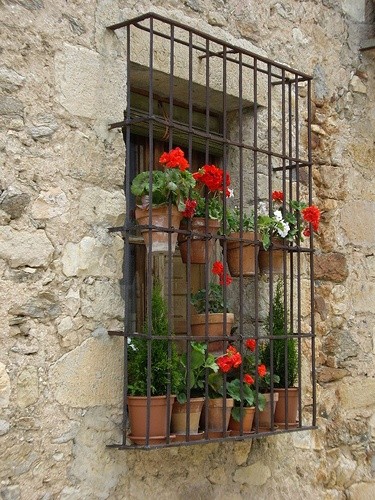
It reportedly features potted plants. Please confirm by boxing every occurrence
[259,276,303,423]
[219,205,260,276]
[126,276,179,437]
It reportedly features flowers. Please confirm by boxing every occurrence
[177,338,280,421]
[258,191,322,249]
[190,261,233,314]
[132,147,234,220]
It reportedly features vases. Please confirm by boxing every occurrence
[229,407,255,431]
[204,398,233,431]
[258,236,288,273]
[191,313,234,351]
[134,205,182,253]
[253,393,279,426]
[171,398,205,434]
[180,216,220,265]
